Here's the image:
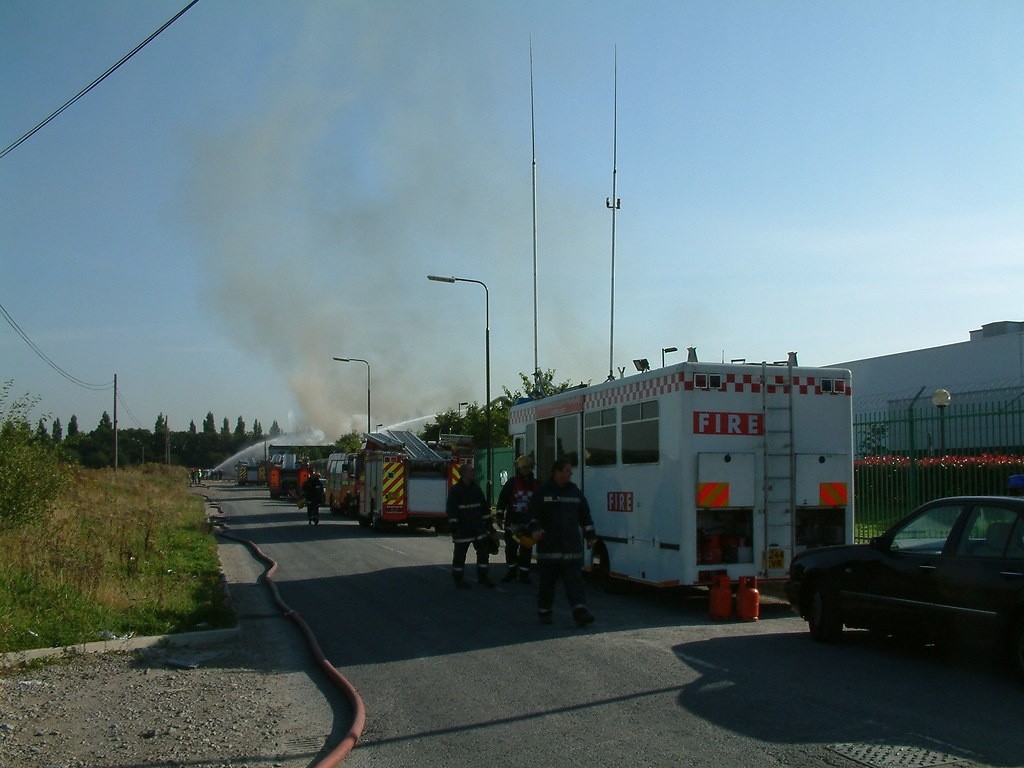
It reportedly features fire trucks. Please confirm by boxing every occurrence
[268,452,312,501]
[234,460,267,487]
[509,362,855,590]
[324,430,475,531]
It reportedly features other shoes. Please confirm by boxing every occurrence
[516,570,531,586]
[572,607,596,628]
[501,568,518,584]
[475,572,497,588]
[537,609,554,625]
[452,573,475,590]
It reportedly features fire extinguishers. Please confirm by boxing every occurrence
[706,573,734,619]
[738,575,761,621]
[697,527,749,565]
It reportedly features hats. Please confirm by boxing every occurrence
[514,455,534,469]
[519,535,537,548]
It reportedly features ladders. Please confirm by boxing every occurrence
[362,432,405,450]
[387,428,444,461]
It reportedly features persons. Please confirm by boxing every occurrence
[496,454,541,585]
[522,459,599,629]
[447,464,494,589]
[211,468,224,481]
[189,468,202,485]
[303,471,327,527]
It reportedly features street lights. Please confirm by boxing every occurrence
[427,274,492,507]
[663,347,676,369]
[332,356,370,436]
[932,388,952,498]
[459,402,468,419]
[376,424,383,435]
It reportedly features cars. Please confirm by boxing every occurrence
[784,496,1024,662]
